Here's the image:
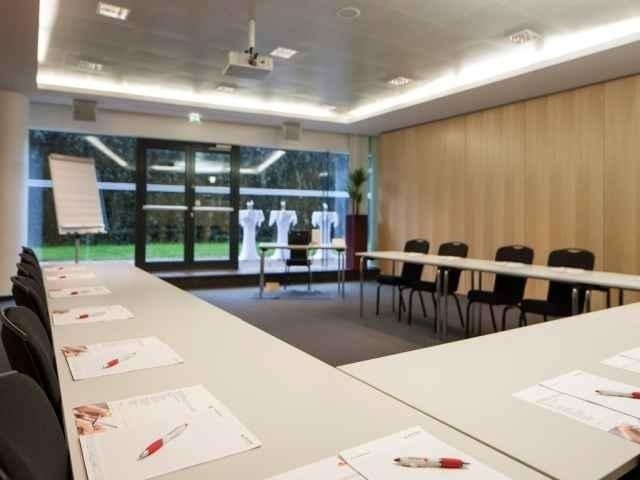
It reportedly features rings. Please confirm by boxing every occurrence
[594,388,640,401]
[393,455,471,469]
[137,421,192,461]
[72,289,89,296]
[59,276,65,278]
[75,309,106,321]
[103,351,136,373]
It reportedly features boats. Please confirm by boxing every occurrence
[284,122,302,141]
[73,98,96,122]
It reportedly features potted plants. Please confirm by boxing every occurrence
[344,167,370,271]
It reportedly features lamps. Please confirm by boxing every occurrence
[283,228,313,290]
[0,244,72,478]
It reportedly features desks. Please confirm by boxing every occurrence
[41,241,640,480]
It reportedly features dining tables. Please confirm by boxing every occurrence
[344,167,370,271]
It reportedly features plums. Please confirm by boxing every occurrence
[221,50,273,80]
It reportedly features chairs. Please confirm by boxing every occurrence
[0,244,72,478]
[283,228,313,290]
